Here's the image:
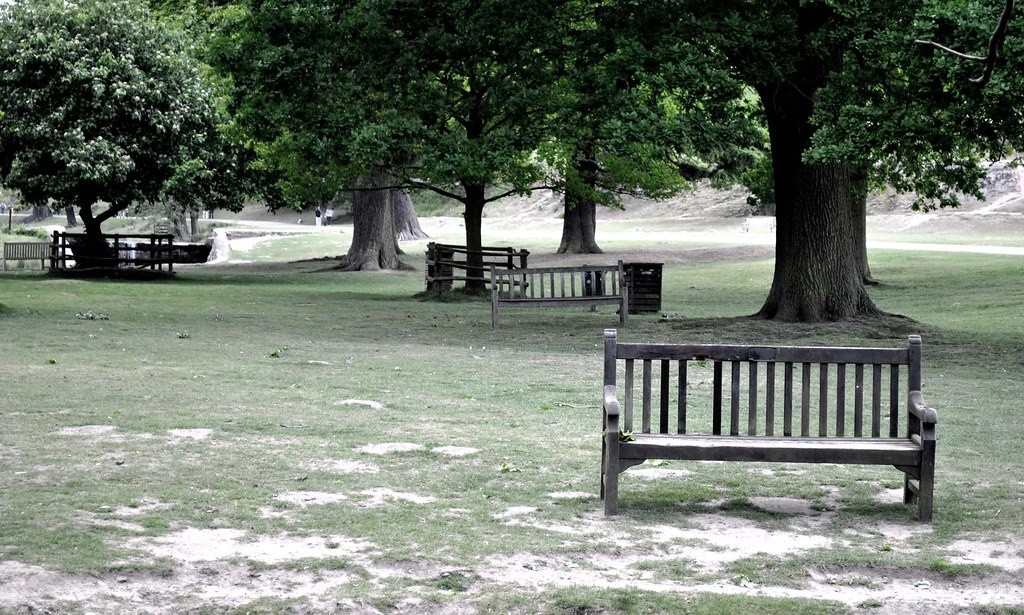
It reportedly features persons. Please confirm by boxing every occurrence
[324,206,334,226]
[118,207,129,219]
[315,204,323,227]
[0,202,6,214]
[202,210,214,219]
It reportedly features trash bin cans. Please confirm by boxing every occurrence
[621,262,665,312]
[583,264,607,295]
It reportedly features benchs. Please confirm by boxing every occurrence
[599,329,939,519]
[3,241,60,271]
[489,260,629,331]
